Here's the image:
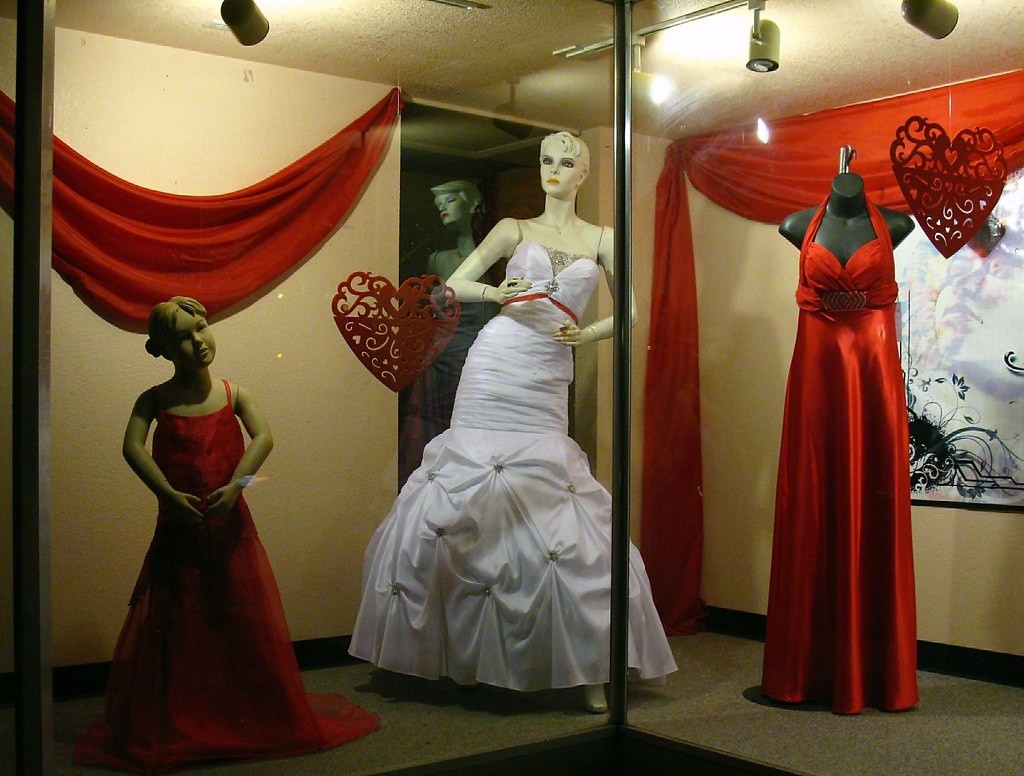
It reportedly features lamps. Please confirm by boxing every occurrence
[901,0,959,40]
[746,0,780,72]
[221,0,270,46]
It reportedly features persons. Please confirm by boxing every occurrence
[69,295,383,776]
[400,155,504,482]
[762,172,919,714]
[348,131,679,712]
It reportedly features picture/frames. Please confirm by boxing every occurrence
[892,168,1024,512]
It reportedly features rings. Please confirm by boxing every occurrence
[508,283,517,287]
